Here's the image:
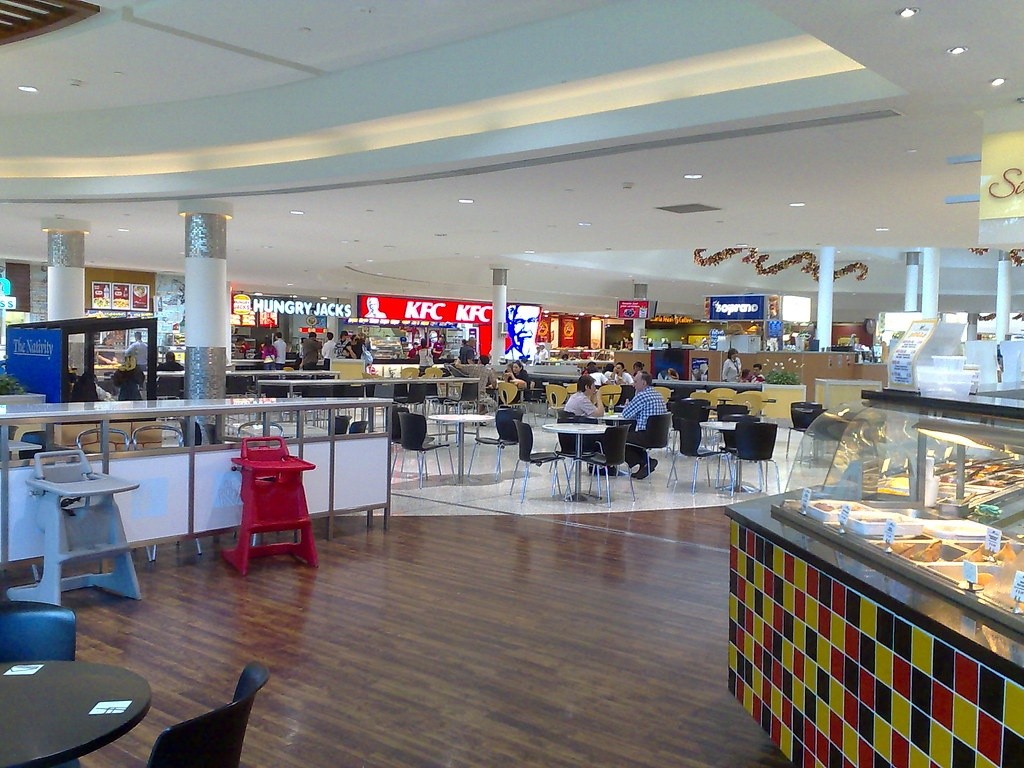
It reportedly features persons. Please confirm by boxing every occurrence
[408,343,419,360]
[321,332,337,371]
[273,332,287,370]
[504,360,531,405]
[432,334,445,360]
[236,337,251,359]
[617,372,667,479]
[470,355,497,410]
[126,332,148,371]
[722,349,765,383]
[533,342,548,365]
[458,340,466,364]
[113,356,145,402]
[337,331,365,359]
[419,339,434,377]
[564,375,624,476]
[157,352,184,396]
[581,362,645,388]
[97,334,117,365]
[461,337,475,364]
[259,338,277,371]
[300,332,322,370]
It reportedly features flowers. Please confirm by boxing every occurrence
[762,357,806,385]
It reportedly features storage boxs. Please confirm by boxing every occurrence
[916,366,976,400]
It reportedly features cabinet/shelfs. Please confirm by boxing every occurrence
[446,327,466,359]
[368,337,403,359]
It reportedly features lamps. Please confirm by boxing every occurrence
[911,418,1005,453]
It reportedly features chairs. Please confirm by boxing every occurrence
[0,601,82,768]
[155,368,828,509]
[147,661,269,768]
[6,450,142,603]
[219,436,319,576]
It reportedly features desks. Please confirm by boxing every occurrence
[8,439,42,582]
[256,378,481,447]
[427,414,496,484]
[717,398,776,405]
[485,387,545,422]
[587,412,636,475]
[702,405,717,411]
[0,661,152,768]
[815,378,882,410]
[142,370,341,382]
[540,423,615,503]
[699,421,759,493]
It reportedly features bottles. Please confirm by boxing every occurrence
[1005,331,1011,341]
[95,285,109,298]
[702,337,708,349]
[608,398,614,415]
[625,399,629,407]
[976,332,995,340]
[115,286,129,299]
[768,321,783,336]
[661,337,669,343]
[997,344,1004,383]
[833,343,852,348]
[641,335,652,345]
[713,299,720,309]
[769,296,780,318]
[709,327,725,346]
[804,336,810,350]
[964,365,980,395]
[704,298,710,318]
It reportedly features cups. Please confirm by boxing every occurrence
[639,308,647,318]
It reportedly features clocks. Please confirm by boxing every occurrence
[864,319,876,334]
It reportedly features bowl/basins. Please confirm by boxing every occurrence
[932,356,967,370]
[917,368,972,402]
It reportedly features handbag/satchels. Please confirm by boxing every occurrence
[425,349,434,366]
[361,346,373,365]
[264,355,275,363]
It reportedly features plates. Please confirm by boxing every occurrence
[134,286,146,297]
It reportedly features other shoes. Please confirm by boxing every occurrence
[589,465,626,476]
[631,459,658,479]
